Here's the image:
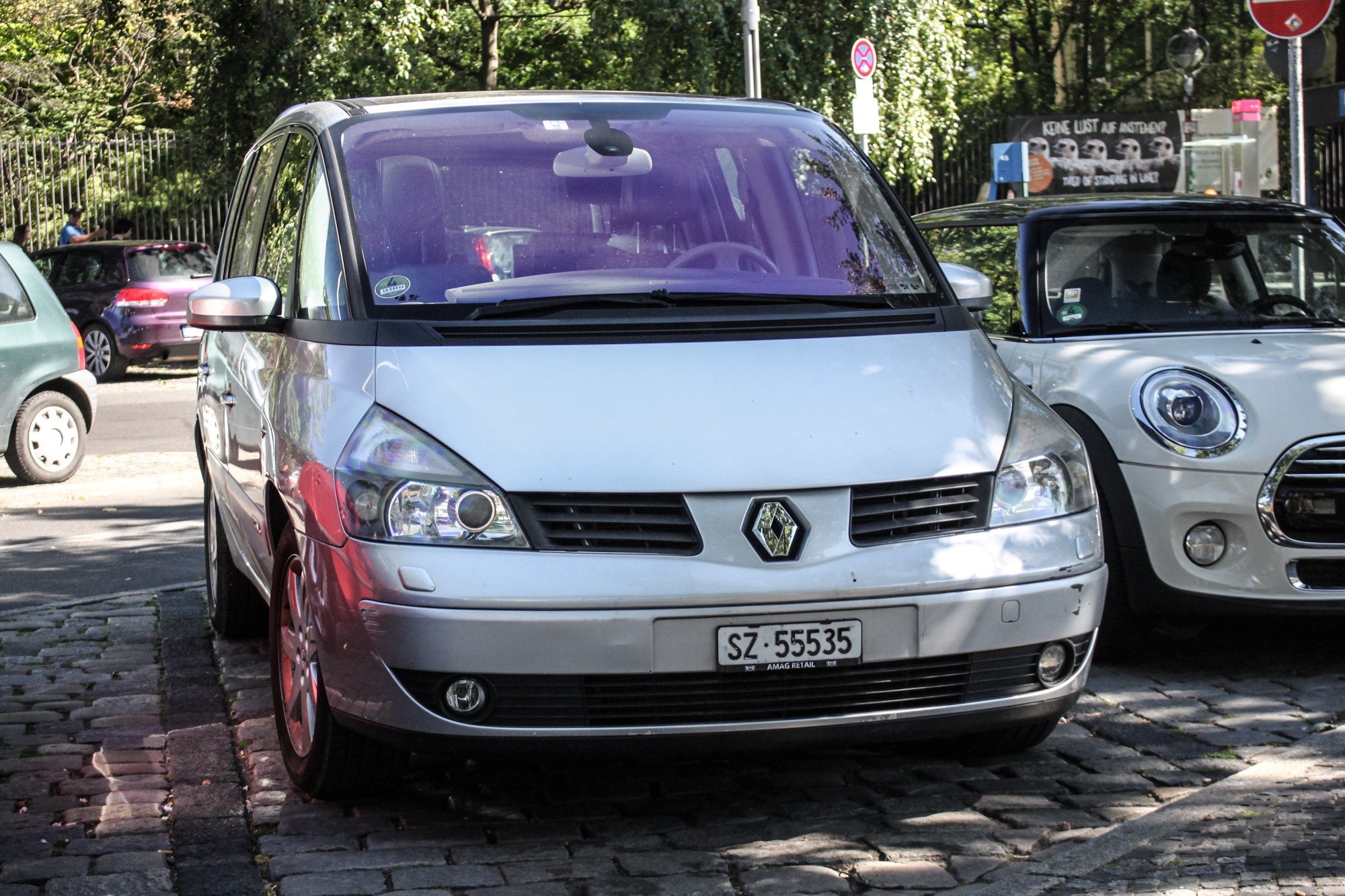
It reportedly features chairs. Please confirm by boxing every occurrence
[1063,250,1228,319]
[361,151,701,298]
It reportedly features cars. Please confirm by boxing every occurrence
[866,190,1345,664]
[0,240,100,485]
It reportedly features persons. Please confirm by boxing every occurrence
[61,207,108,283]
[11,222,31,253]
[109,218,133,241]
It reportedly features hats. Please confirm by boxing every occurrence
[68,207,82,214]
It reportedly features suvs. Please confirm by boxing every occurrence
[28,240,218,385]
[184,87,1110,805]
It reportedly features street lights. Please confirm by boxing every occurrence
[1165,26,1212,193]
